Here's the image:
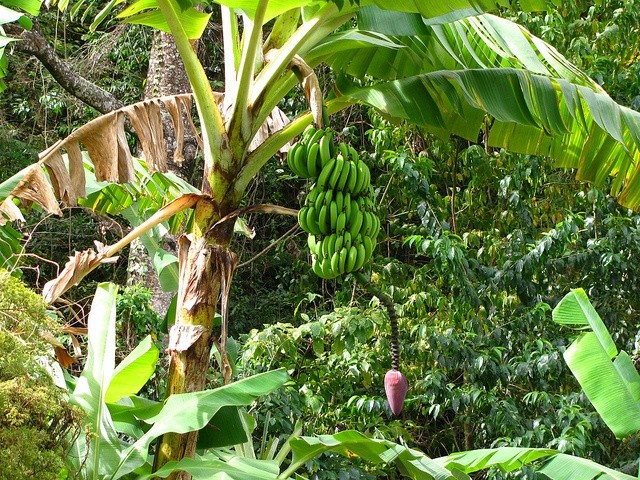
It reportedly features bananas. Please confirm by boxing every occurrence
[289,120,380,279]
